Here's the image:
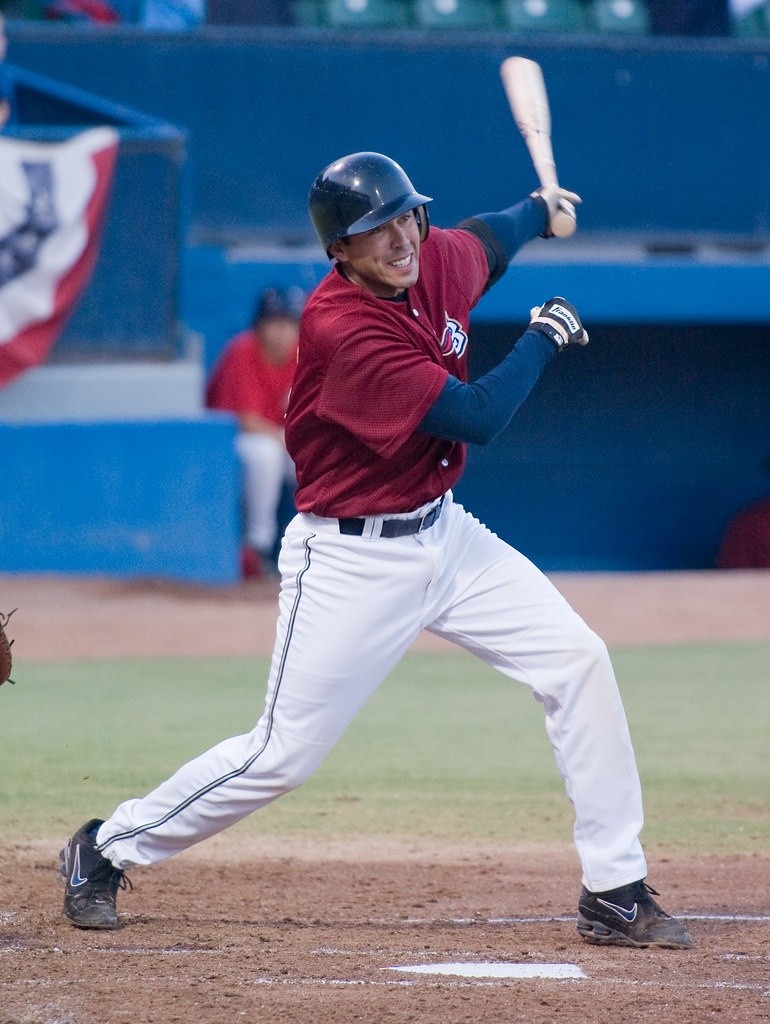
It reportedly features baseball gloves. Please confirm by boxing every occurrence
[0,608,19,687]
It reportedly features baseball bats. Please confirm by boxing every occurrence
[500,56,576,238]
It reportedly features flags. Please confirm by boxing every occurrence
[0,120,117,389]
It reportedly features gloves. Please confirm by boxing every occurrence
[528,297,589,354]
[530,183,583,240]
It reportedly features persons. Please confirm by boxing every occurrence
[51,150,694,949]
[204,285,305,581]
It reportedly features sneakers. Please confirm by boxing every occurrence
[575,881,694,949]
[57,819,133,928]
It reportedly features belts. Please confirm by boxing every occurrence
[339,496,445,537]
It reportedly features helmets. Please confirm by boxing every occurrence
[307,153,433,260]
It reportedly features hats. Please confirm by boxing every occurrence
[250,291,307,321]
